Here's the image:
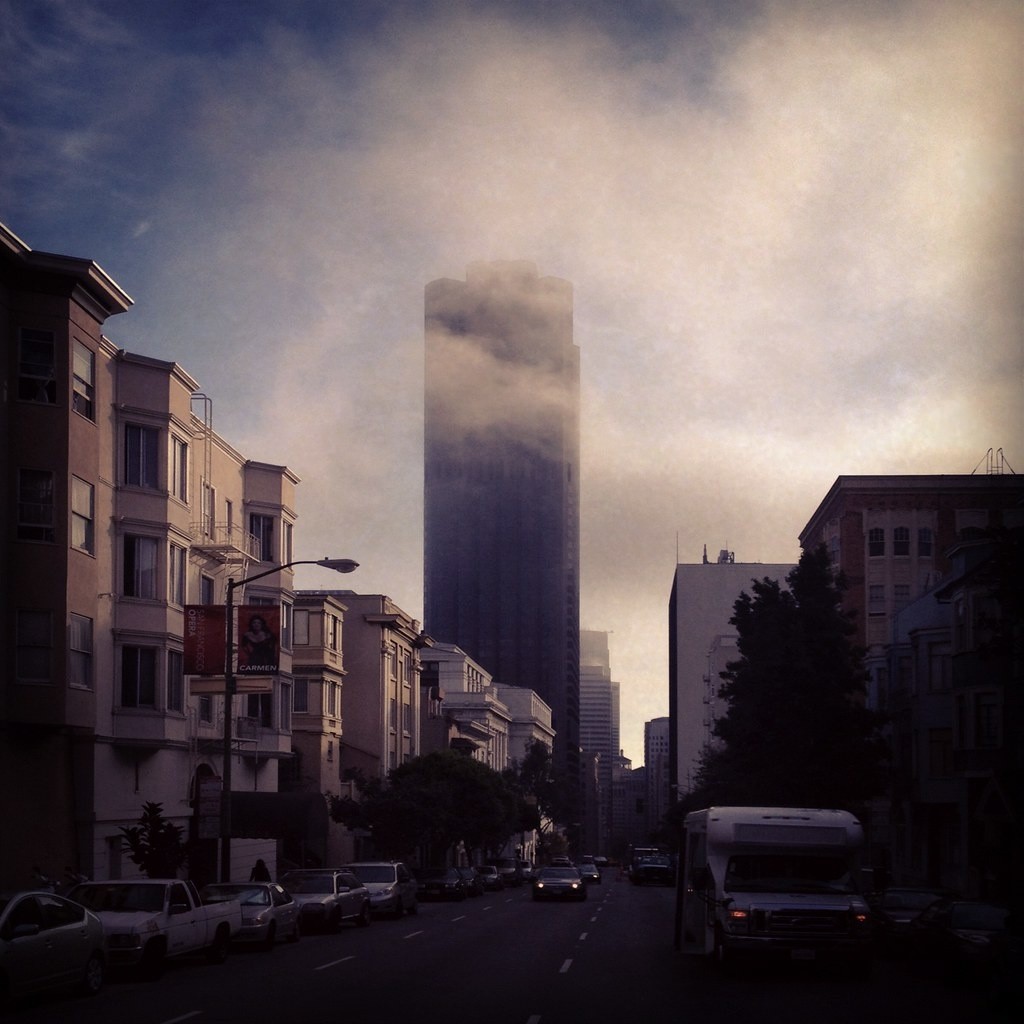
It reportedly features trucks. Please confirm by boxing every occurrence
[677,802,893,985]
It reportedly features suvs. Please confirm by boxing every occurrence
[339,858,419,921]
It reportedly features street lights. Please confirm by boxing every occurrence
[219,557,359,883]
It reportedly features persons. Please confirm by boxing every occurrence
[249,859,272,883]
[33,866,80,894]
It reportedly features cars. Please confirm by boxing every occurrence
[196,881,304,952]
[898,894,1022,981]
[494,855,619,887]
[411,865,469,902]
[273,867,372,935]
[452,864,487,899]
[529,864,588,903]
[0,889,107,1003]
[577,862,603,886]
[862,880,967,950]
[477,864,507,892]
[629,847,676,886]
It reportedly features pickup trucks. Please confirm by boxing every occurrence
[64,878,244,983]
